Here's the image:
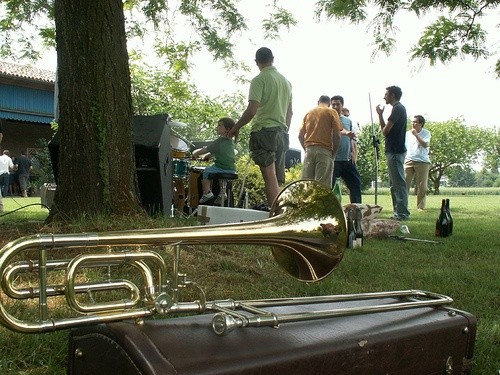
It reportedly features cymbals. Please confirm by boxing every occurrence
[166,116,187,130]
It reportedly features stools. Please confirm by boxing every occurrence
[208,172,238,207]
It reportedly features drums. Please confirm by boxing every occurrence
[190,165,235,209]
[168,131,188,179]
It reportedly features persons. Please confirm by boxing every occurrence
[298,95,342,190]
[331,95,361,204]
[225,46,293,212]
[376,86,410,222]
[192,117,236,203]
[0,150,15,197]
[404,115,431,212]
[8,155,20,196]
[15,152,32,198]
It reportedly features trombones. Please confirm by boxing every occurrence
[0,178,453,336]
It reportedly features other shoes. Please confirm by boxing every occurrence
[214,192,227,204]
[198,190,214,204]
[391,213,410,221]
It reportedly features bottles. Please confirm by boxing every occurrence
[446,199,453,234]
[354,209,363,247]
[436,199,449,238]
[347,208,356,249]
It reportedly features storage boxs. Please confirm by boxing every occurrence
[67,296,477,375]
